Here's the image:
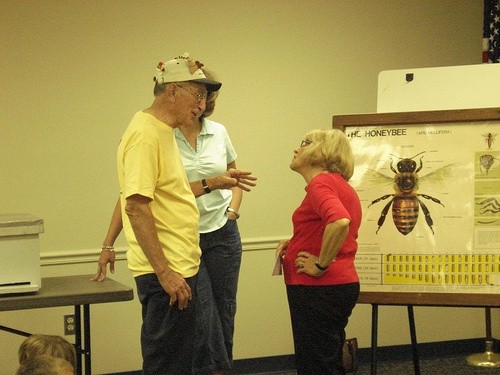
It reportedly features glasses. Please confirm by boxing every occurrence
[300,140,310,146]
[175,82,204,103]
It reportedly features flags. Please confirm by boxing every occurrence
[482,0,500,64]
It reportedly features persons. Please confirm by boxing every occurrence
[89,69,243,367]
[117,55,257,375]
[16,354,73,375]
[275,128,364,375]
[18,334,76,375]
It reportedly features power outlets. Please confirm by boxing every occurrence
[64,314,76,337]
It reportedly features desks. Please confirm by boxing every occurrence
[0,270,134,375]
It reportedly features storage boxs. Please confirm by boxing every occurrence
[0,214,46,295]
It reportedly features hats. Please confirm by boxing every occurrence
[152,56,222,91]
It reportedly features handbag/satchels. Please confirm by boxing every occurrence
[342,338,359,373]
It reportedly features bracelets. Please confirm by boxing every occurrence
[228,208,240,219]
[314,258,328,271]
[201,179,211,193]
[101,245,114,252]
